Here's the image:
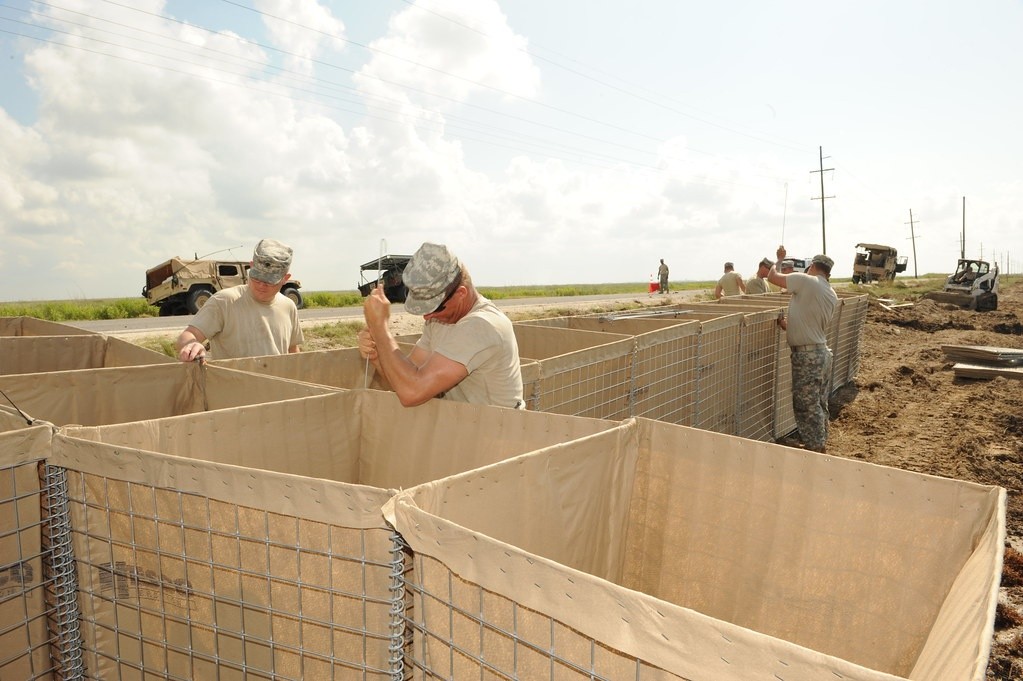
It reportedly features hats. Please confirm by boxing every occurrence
[781,261,795,270]
[763,258,774,267]
[725,262,733,268]
[402,243,461,315]
[248,239,292,285]
[803,254,833,277]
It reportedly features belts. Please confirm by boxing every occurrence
[791,343,828,353]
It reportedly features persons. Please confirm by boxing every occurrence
[959,267,976,286]
[715,262,745,299]
[764,261,794,292]
[657,259,669,294]
[175,238,305,366]
[746,257,774,294]
[767,245,837,454]
[358,243,526,410]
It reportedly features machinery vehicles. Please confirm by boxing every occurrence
[920,259,998,312]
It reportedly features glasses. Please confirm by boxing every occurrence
[435,283,460,313]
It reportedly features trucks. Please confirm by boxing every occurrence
[783,256,812,274]
[852,242,908,284]
[358,253,415,304]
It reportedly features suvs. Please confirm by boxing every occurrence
[143,255,303,315]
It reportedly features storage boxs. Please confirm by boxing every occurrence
[0,287,1010,681]
